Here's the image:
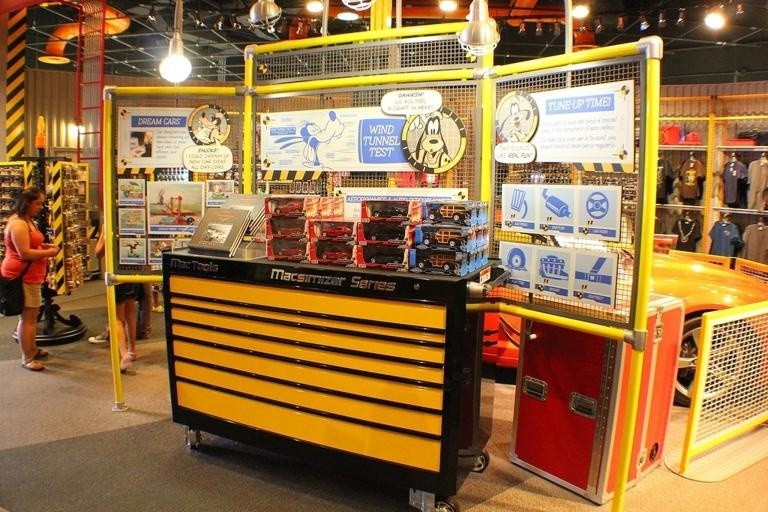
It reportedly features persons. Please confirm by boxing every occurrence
[0,187,60,372]
[87,224,165,373]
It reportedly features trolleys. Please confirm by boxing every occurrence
[157,247,512,511]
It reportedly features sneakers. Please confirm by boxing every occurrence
[88,335,107,344]
[120,353,136,373]
[153,305,164,312]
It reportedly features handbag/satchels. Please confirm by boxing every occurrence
[0,275,25,316]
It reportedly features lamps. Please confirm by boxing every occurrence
[457,0,502,57]
[248,0,283,33]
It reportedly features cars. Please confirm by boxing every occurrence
[482,219,768,409]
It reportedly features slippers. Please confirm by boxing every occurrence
[34,349,47,359]
[22,360,44,371]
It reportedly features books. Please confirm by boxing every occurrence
[188,194,265,258]
